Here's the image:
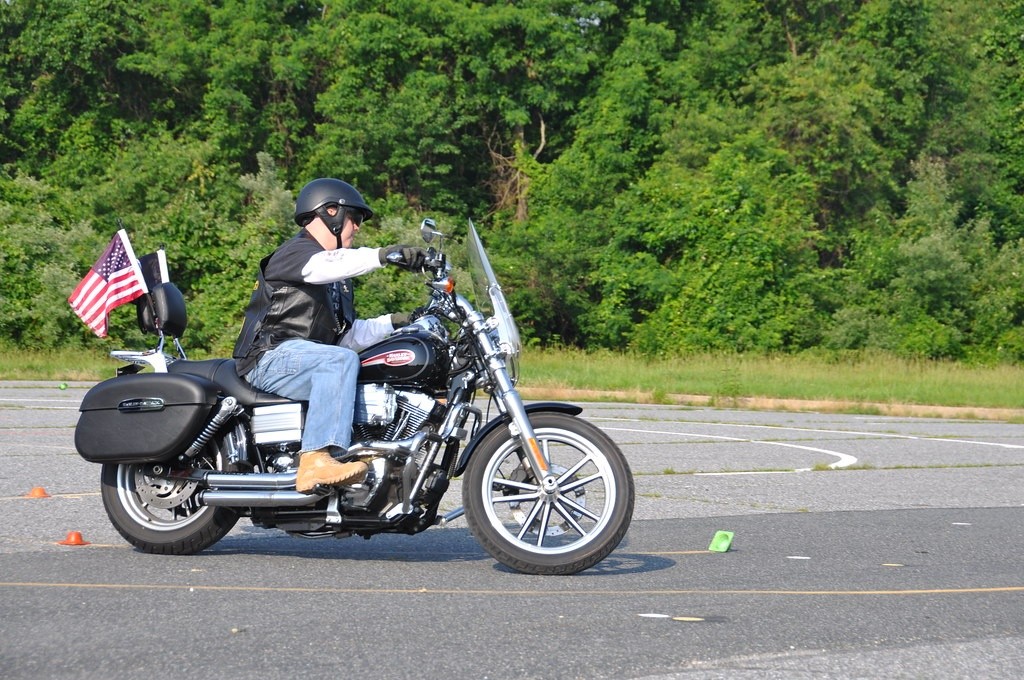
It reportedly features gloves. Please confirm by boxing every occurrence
[378,245,430,274]
[391,312,415,329]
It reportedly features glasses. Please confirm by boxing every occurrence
[347,210,363,227]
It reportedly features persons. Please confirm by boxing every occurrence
[232,177,430,495]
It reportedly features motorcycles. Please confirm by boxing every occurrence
[74,220,635,577]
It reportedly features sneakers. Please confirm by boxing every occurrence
[296,452,368,494]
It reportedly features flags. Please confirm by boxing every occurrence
[68,229,149,340]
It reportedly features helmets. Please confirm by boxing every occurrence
[295,178,373,227]
